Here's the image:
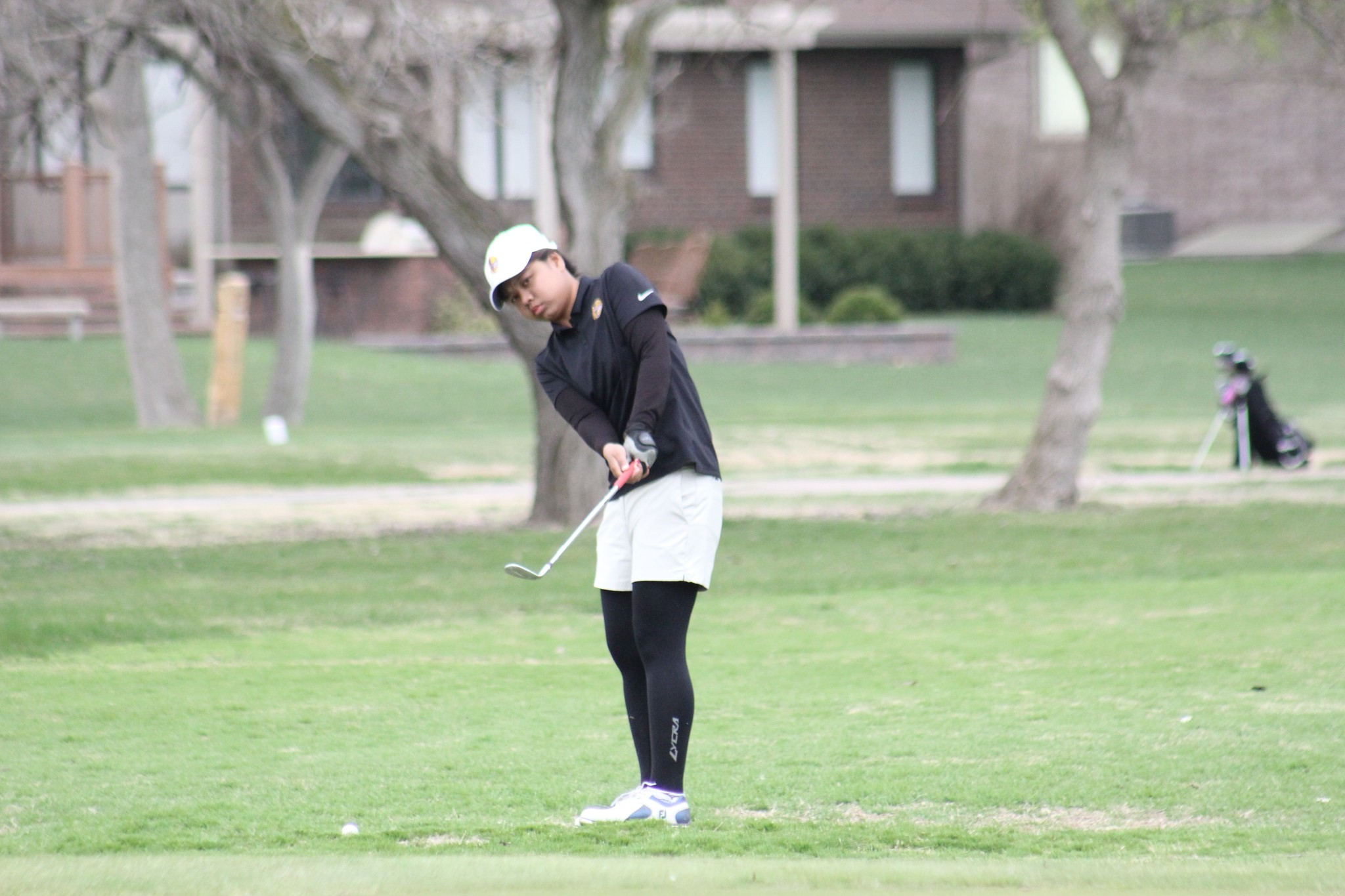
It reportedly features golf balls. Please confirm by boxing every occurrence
[263,415,290,445]
[341,824,359,835]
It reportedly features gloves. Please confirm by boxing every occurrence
[623,430,659,475]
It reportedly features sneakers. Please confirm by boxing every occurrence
[578,782,691,827]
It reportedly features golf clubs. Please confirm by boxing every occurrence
[504,458,640,581]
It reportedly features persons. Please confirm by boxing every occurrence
[484,222,725,831]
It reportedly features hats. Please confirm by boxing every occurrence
[483,223,558,312]
